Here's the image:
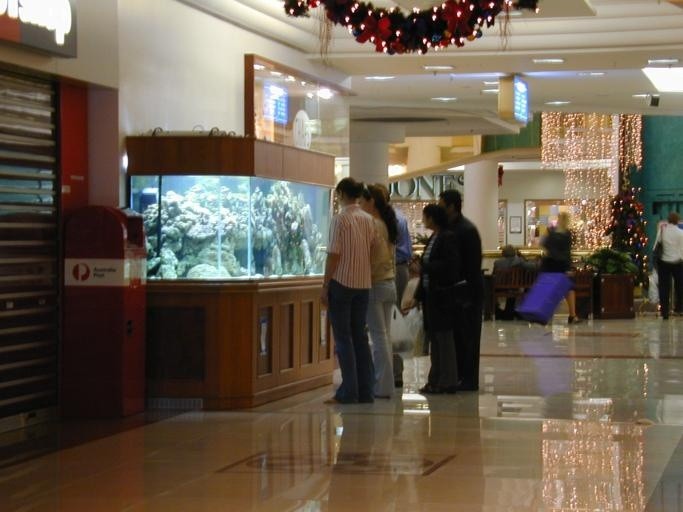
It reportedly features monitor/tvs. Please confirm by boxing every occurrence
[262,81,288,126]
[498,75,529,127]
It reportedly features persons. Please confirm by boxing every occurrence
[488,243,527,322]
[652,212,682,321]
[412,203,459,395]
[319,177,379,404]
[373,184,411,389]
[537,211,582,325]
[356,181,397,399]
[435,188,487,392]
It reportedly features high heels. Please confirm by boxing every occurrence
[566,315,579,326]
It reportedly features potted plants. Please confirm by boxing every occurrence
[586,247,639,319]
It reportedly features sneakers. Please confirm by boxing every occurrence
[325,396,373,405]
[417,380,480,394]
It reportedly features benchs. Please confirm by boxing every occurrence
[486,266,593,323]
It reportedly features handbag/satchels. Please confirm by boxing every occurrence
[652,242,663,270]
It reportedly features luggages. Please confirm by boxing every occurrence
[514,265,575,325]
